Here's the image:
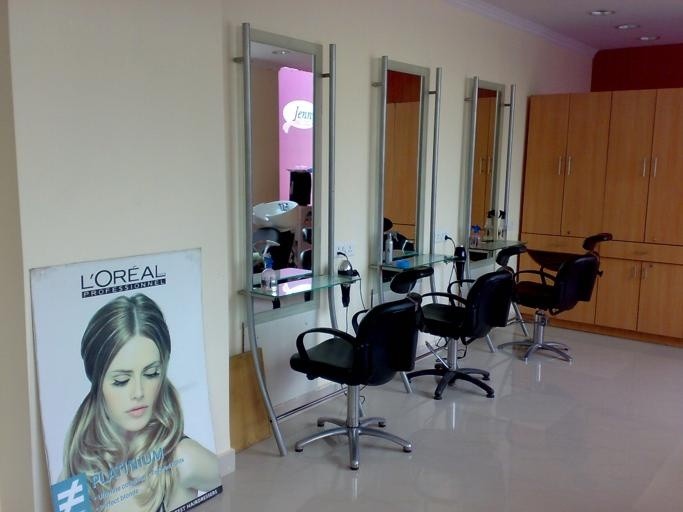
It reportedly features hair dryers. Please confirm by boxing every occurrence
[337,260,359,309]
[454,243,468,289]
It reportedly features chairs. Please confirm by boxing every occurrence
[290,266,429,470]
[300,228,312,300]
[406,244,526,400]
[496,233,613,363]
[253,228,295,309]
[383,217,414,284]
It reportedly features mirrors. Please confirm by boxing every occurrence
[383,60,430,291]
[458,79,505,272]
[249,29,323,325]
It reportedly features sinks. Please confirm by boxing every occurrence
[252,200,301,232]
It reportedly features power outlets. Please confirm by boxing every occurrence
[334,244,355,258]
[435,232,448,241]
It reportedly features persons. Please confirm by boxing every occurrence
[58,292,220,512]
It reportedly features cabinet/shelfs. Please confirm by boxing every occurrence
[383,101,419,240]
[596,87,683,346]
[472,97,496,235]
[519,86,612,331]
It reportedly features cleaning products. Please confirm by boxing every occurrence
[499,211,507,241]
[481,209,496,241]
[385,233,394,263]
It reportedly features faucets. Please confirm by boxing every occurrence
[277,201,289,211]
